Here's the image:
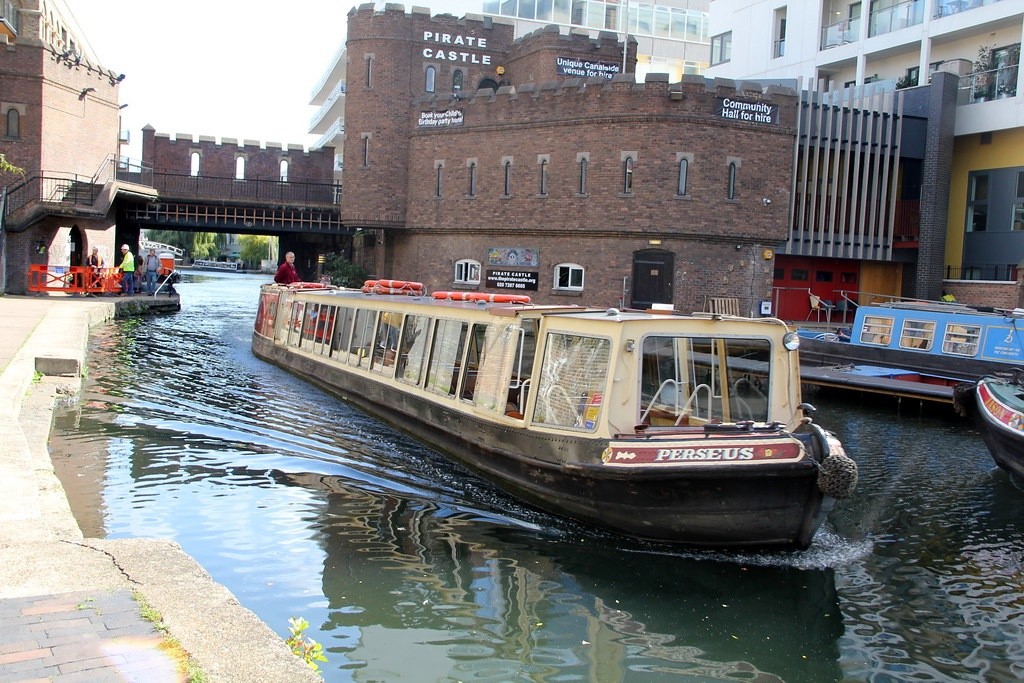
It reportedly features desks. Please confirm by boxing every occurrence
[947,0,969,15]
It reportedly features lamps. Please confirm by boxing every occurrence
[83,87,99,94]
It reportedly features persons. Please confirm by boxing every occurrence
[86,243,160,297]
[274,251,303,284]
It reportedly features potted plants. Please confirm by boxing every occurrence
[974,90,990,103]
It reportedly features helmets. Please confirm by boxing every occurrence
[120,243,130,250]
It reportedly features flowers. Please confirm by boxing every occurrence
[998,84,1016,95]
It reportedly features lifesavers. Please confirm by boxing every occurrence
[431,289,532,305]
[359,277,425,298]
[289,280,329,289]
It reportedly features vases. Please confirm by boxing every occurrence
[1001,93,1012,99]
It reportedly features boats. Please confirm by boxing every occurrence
[951,367,1024,478]
[788,297,1024,386]
[249,279,860,551]
[192,259,249,274]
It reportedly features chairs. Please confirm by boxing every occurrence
[805,296,828,323]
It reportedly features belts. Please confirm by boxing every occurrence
[148,270,156,272]
